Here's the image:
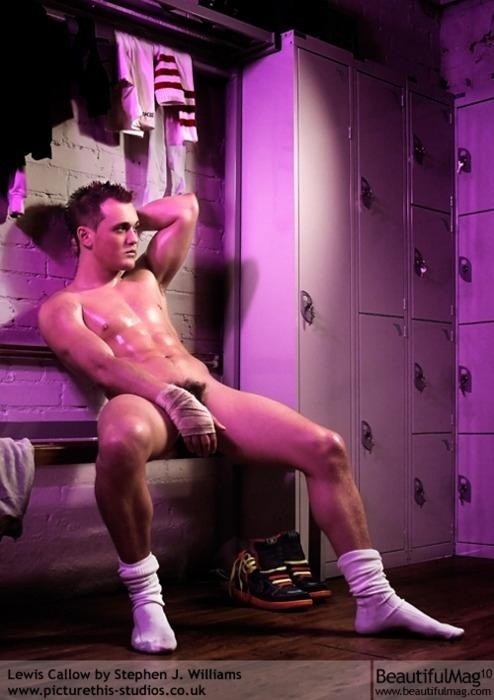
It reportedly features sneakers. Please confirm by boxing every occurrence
[224,539,313,610]
[269,531,333,598]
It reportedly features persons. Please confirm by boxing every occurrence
[37,181,464,655]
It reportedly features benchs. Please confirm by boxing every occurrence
[0,343,244,466]
